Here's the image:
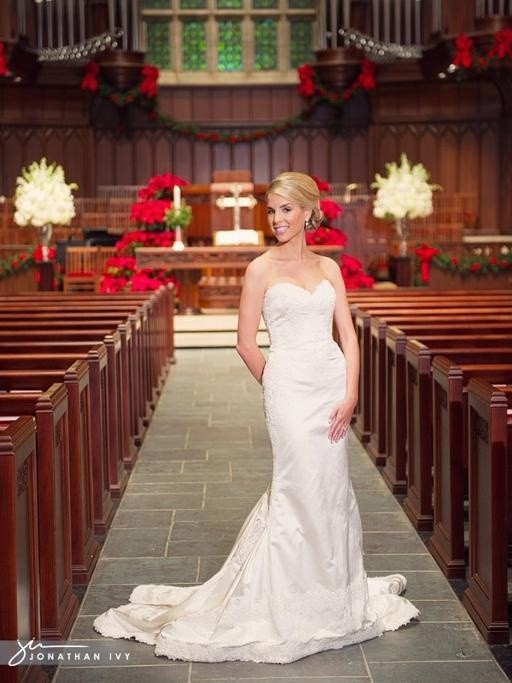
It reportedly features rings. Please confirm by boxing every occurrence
[343,426,349,433]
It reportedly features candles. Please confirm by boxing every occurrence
[173,183,182,221]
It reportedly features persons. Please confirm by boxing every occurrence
[94,172,421,665]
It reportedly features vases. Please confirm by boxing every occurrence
[386,254,416,284]
[1,238,68,294]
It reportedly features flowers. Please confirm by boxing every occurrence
[305,176,375,292]
[100,175,194,298]
[11,159,84,259]
[369,152,434,259]
